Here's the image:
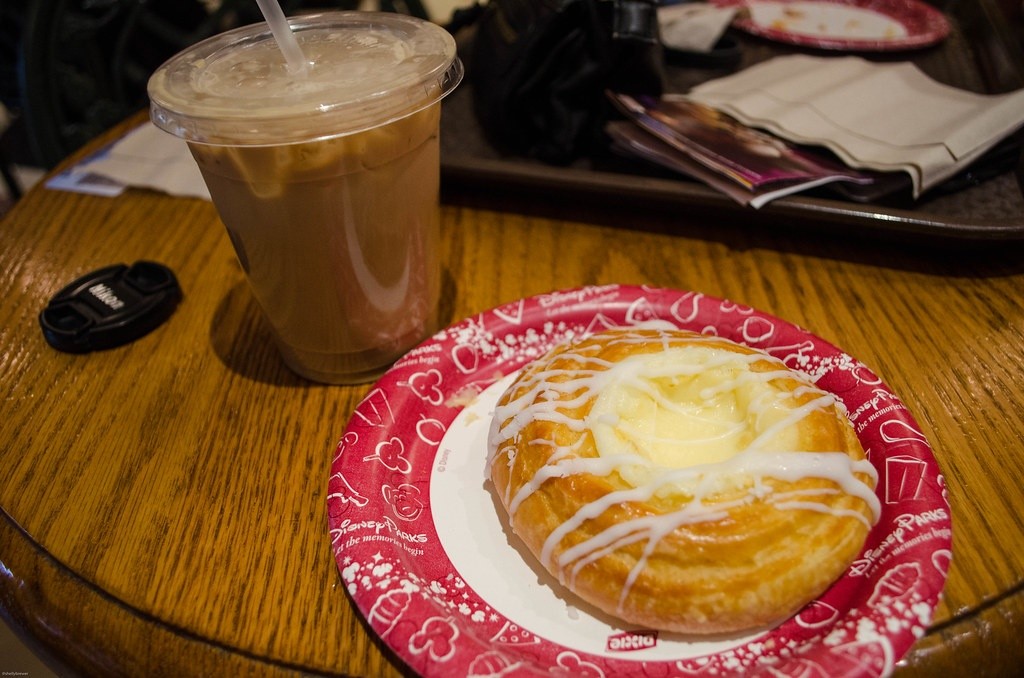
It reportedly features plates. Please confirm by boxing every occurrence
[711,0,955,58]
[327,283,955,678]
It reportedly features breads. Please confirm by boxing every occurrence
[487,330,879,632]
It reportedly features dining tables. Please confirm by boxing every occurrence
[0,109,1023,678]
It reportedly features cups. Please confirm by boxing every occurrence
[147,12,466,386]
